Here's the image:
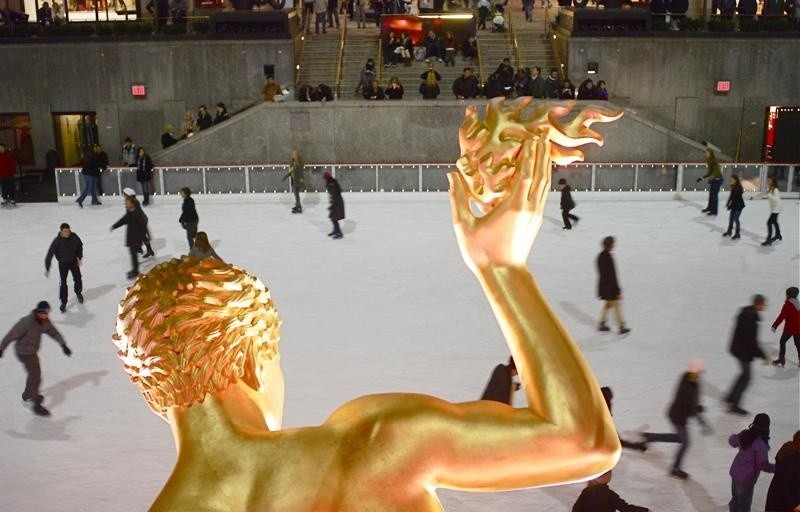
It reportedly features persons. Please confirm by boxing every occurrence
[280,1,340,34]
[348,1,433,29]
[749,175,782,247]
[43,222,84,315]
[1,302,72,417]
[641,357,714,480]
[356,58,480,100]
[592,386,648,453]
[559,1,799,35]
[558,177,580,231]
[770,286,800,368]
[478,0,535,29]
[764,429,799,511]
[109,196,147,281]
[723,173,747,240]
[727,414,777,512]
[481,355,523,404]
[1,102,230,215]
[696,148,724,215]
[122,187,156,259]
[262,75,333,101]
[595,236,630,336]
[177,187,199,253]
[188,231,224,260]
[281,150,303,213]
[384,29,476,67]
[487,58,608,100]
[718,293,770,414]
[321,170,346,239]
[572,469,651,512]
[114,93,625,512]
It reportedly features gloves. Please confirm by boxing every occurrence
[63,346,70,354]
[697,178,702,182]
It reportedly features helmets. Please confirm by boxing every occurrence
[123,187,136,196]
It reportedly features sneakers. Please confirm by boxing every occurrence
[92,202,101,204]
[328,232,342,238]
[563,225,571,229]
[773,359,785,365]
[139,248,154,257]
[60,302,66,311]
[673,470,688,477]
[727,394,746,415]
[0,198,16,207]
[761,234,782,245]
[22,392,48,415]
[75,289,83,303]
[724,231,740,239]
[599,325,629,333]
[127,270,138,274]
[77,199,83,208]
[703,208,717,215]
[292,207,301,213]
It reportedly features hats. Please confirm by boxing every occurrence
[787,287,799,299]
[38,300,50,313]
[324,172,331,178]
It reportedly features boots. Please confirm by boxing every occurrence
[143,192,149,205]
